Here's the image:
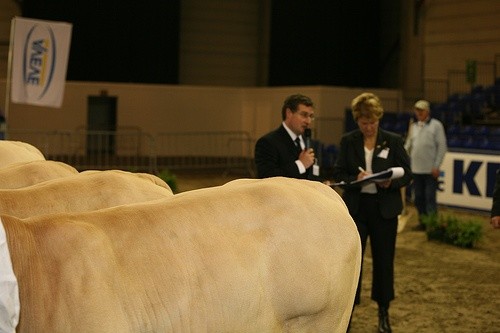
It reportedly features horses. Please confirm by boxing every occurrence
[0,170,177,217]
[1,137,46,168]
[0,175,363,333]
[1,159,80,188]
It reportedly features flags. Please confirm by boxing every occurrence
[11,18,72,108]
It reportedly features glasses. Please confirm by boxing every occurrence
[292,110,318,121]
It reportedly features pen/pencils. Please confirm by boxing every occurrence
[358,166,365,172]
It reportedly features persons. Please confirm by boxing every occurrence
[332,92,411,333]
[254,95,319,181]
[491,170,500,228]
[404,101,446,217]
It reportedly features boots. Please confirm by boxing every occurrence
[377,303,392,333]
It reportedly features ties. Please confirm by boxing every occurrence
[294,137,302,160]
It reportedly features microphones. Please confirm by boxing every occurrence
[305,129,311,150]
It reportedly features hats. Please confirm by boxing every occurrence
[414,100,430,111]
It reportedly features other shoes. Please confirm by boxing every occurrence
[411,222,426,231]
[426,223,438,240]
